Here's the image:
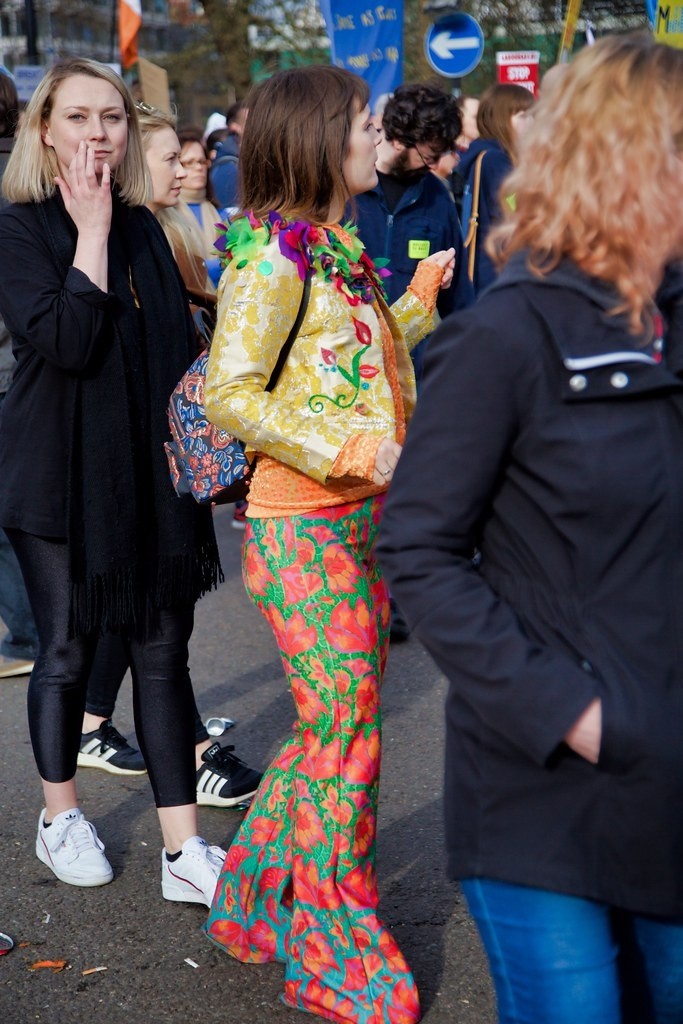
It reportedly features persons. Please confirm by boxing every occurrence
[202,67,457,1024]
[339,84,535,641]
[374,36,683,1024]
[0,56,228,912]
[1,69,40,678]
[178,104,247,528]
[76,103,265,808]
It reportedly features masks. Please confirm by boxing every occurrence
[397,165,430,186]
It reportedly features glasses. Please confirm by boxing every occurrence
[136,102,155,115]
[180,159,212,169]
[413,143,441,165]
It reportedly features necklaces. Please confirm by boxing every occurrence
[262,215,385,305]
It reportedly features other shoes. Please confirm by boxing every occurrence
[0,655,35,678]
[231,500,248,529]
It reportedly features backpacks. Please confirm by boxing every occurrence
[163,265,311,504]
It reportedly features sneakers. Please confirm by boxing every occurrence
[161,836,228,909]
[77,718,147,775]
[196,742,263,806]
[36,807,114,887]
